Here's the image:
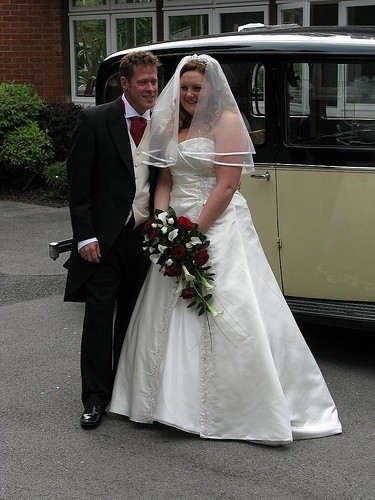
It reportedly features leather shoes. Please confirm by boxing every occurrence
[78,407,110,430]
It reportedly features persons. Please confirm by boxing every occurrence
[134,54,258,434]
[64,51,242,428]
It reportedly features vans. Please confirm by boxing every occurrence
[48,25,375,323]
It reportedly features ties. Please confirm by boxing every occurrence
[130,114,148,144]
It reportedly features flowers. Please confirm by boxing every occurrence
[142,207,225,319]
